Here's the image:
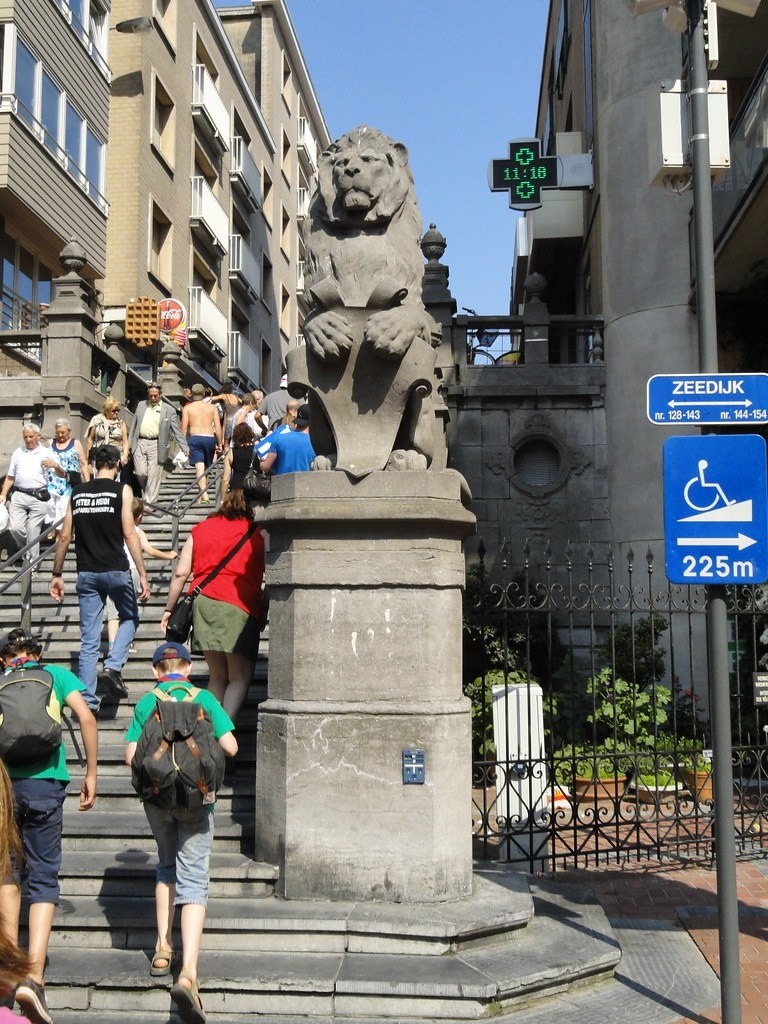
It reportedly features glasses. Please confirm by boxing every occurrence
[111,409,121,413]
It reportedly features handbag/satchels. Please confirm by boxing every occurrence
[66,471,82,486]
[245,467,270,495]
[36,491,51,502]
[164,590,197,645]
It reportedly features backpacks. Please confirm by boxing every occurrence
[0,665,88,772]
[135,686,228,814]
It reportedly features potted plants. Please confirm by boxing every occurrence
[630,772,684,804]
[572,771,626,810]
[619,731,703,781]
[681,754,714,806]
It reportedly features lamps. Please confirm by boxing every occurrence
[109,16,153,34]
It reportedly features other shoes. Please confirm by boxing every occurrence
[14,975,53,1024]
[30,570,38,578]
[48,530,56,541]
[200,497,212,506]
[99,668,131,701]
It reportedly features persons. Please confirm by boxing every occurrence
[86,396,129,481]
[125,641,238,1024]
[182,383,222,504]
[49,444,151,717]
[104,498,178,653]
[220,373,317,504]
[0,628,98,1023]
[0,422,66,578]
[128,381,190,515]
[44,418,90,543]
[161,486,270,721]
[204,382,242,462]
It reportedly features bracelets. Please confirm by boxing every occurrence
[54,464,60,470]
[53,572,62,576]
[164,610,172,612]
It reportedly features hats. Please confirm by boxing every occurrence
[279,373,289,389]
[148,643,192,662]
[173,451,189,469]
[0,626,40,651]
[192,383,207,402]
[292,404,310,427]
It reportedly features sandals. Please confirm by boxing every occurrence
[168,970,208,1024]
[151,949,178,979]
